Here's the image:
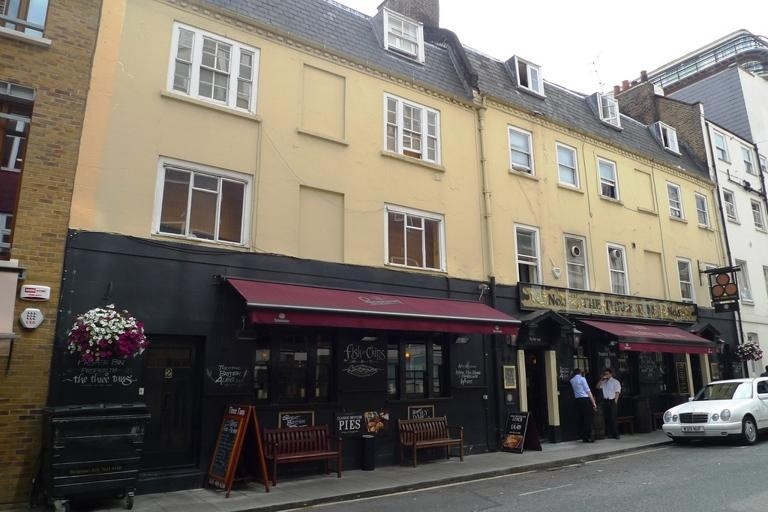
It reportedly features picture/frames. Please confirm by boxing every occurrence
[406,404,435,420]
[277,410,315,428]
[502,365,518,389]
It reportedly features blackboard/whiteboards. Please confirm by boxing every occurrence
[207,406,251,488]
[502,412,530,452]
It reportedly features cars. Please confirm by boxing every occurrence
[661,376,768,447]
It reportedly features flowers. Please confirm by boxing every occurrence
[735,339,763,361]
[67,309,150,364]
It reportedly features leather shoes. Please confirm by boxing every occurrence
[583,438,593,443]
[608,434,620,439]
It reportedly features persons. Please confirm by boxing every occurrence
[760,365,768,377]
[596,369,622,439]
[569,368,598,443]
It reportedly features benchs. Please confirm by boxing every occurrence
[258,424,344,486]
[397,415,465,468]
